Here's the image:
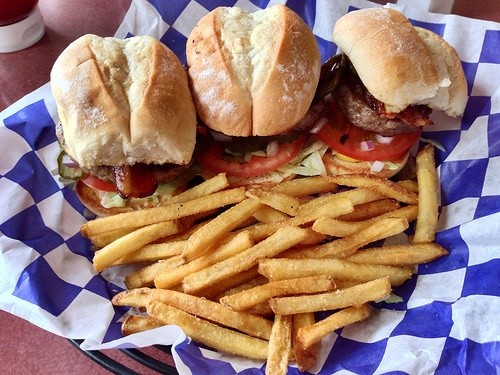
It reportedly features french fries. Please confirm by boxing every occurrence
[80,144,448,375]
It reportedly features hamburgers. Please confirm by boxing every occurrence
[185,3,320,189]
[320,6,468,177]
[49,33,198,216]
